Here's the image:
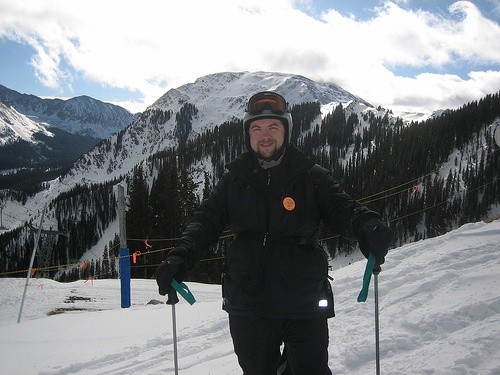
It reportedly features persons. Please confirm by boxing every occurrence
[154,90,396,375]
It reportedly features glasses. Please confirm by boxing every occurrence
[249,92,288,115]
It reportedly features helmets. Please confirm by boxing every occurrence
[243,104,292,151]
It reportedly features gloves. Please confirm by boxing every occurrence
[155,254,186,296]
[358,217,393,264]
